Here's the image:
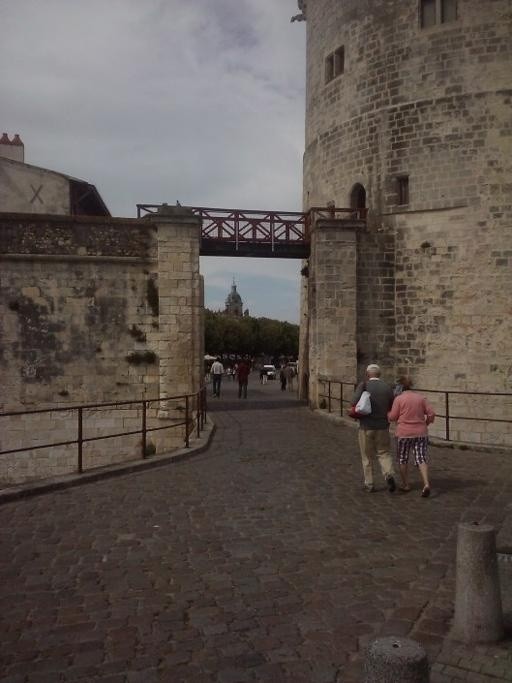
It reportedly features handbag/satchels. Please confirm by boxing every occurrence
[348,390,372,420]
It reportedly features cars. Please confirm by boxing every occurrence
[264,364,277,379]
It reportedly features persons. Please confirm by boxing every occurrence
[387,375,436,499]
[234,359,249,400]
[350,363,397,495]
[259,360,294,394]
[210,357,224,399]
[226,362,238,382]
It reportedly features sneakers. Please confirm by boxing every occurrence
[422,484,430,497]
[386,474,395,493]
